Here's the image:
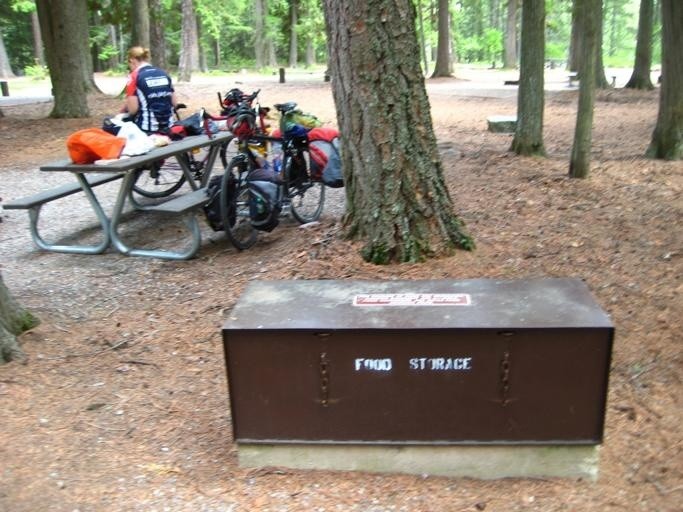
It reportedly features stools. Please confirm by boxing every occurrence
[137,183,220,261]
[1,173,124,250]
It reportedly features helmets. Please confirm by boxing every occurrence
[227,103,256,138]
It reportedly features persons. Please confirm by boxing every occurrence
[122,46,177,133]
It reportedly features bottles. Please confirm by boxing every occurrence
[256,198,266,216]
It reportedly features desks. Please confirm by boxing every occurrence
[2,128,244,261]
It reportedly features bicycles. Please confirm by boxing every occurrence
[203,88,326,251]
[123,102,279,198]
